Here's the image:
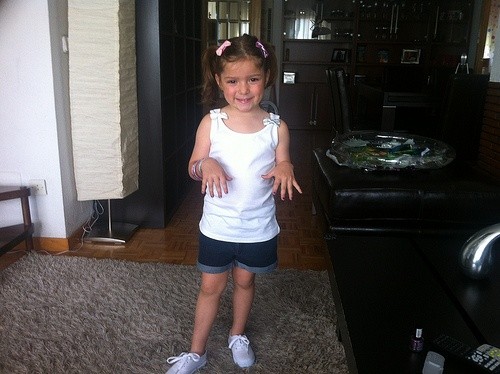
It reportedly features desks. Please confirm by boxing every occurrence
[324,234,500,374]
[358,82,452,143]
[311,147,498,234]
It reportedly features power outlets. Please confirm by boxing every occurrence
[29,180,47,195]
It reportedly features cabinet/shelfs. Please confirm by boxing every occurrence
[95,0,202,229]
[280,0,476,131]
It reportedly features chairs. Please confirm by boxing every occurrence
[323,66,354,136]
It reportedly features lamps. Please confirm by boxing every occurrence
[64,0,140,244]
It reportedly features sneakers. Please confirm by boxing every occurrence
[228,334,256,367]
[164,352,207,374]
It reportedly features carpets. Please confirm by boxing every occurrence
[0,251,347,374]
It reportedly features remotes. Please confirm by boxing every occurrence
[432,334,500,374]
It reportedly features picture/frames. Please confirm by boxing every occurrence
[403,49,421,64]
[331,49,347,63]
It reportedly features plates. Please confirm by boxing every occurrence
[330,132,456,167]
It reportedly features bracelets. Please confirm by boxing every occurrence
[189,158,207,181]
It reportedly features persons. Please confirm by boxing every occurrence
[455,54,470,79]
[166,33,303,374]
